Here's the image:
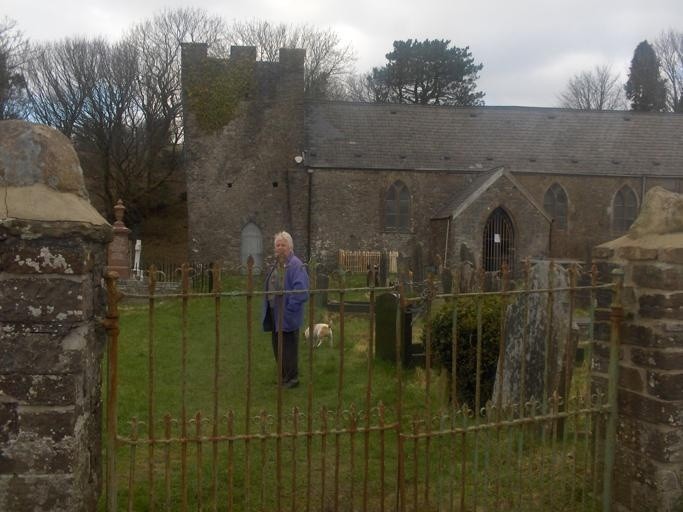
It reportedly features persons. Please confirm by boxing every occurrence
[262,230,309,389]
[207,261,213,292]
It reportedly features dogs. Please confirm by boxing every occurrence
[303,319,334,349]
